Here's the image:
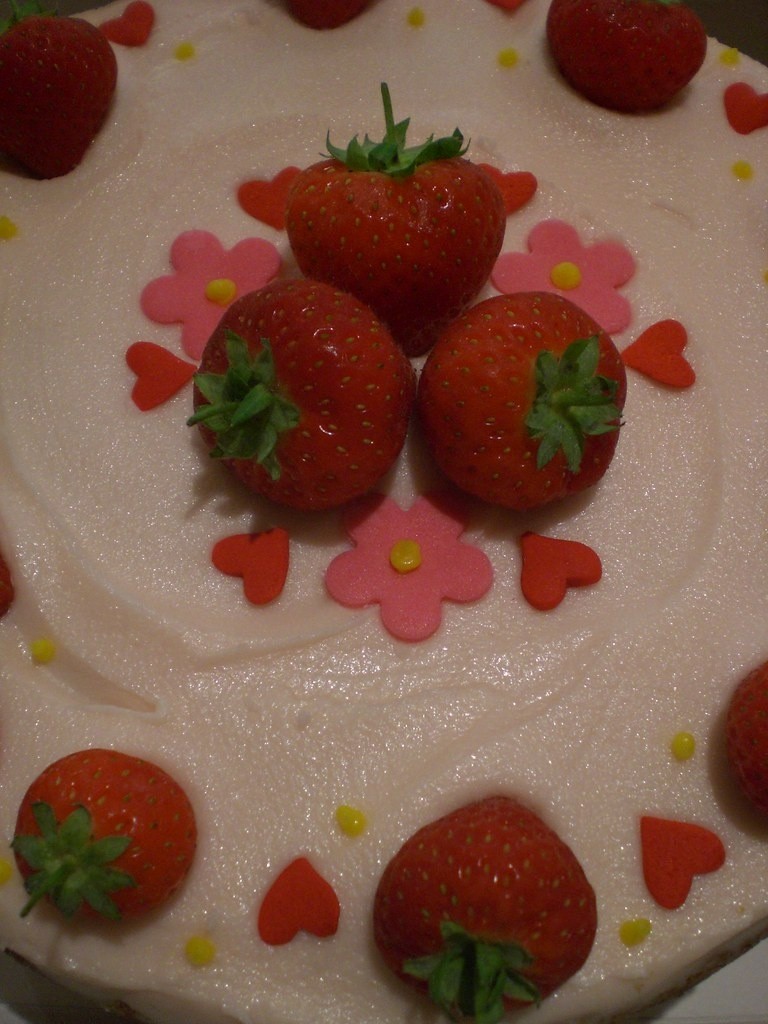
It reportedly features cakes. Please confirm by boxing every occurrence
[0,0,768,1024]
